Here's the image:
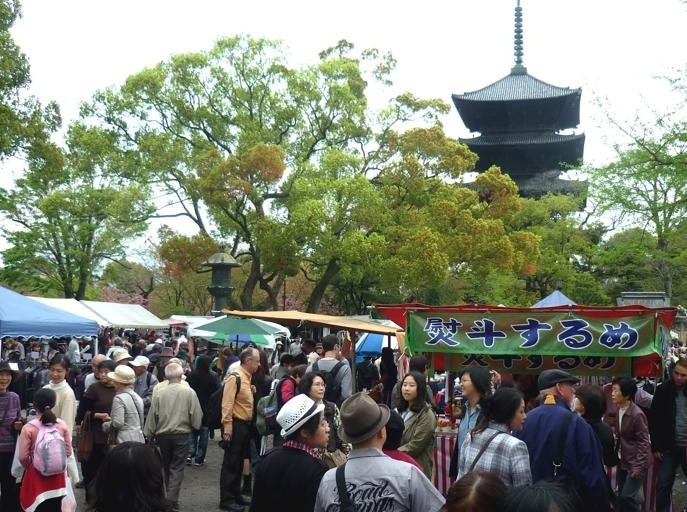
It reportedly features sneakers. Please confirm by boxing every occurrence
[75,478,87,488]
[186,456,207,467]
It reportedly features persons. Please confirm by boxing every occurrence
[442,339,687,511]
[219,335,446,511]
[1,332,218,511]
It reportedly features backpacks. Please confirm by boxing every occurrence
[29,421,67,476]
[256,378,283,436]
[312,361,344,402]
[205,372,240,429]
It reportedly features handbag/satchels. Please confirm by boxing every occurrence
[103,426,118,456]
[78,411,94,461]
[320,449,347,469]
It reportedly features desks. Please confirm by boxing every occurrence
[429,417,459,496]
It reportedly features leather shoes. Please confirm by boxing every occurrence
[226,502,246,512]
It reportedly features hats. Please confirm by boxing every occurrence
[152,338,189,357]
[275,393,325,436]
[106,339,150,384]
[537,369,580,391]
[276,340,284,345]
[337,392,391,444]
[0,361,18,379]
